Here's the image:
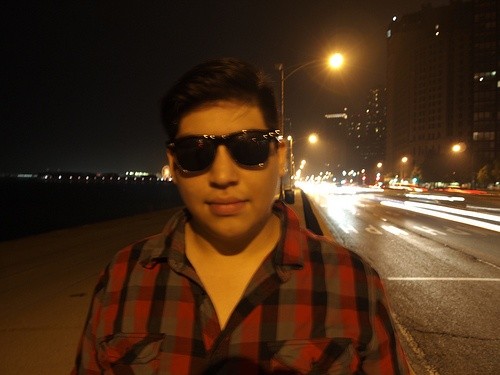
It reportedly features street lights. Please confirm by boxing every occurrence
[279,52,344,201]
[451,144,475,190]
[291,132,319,179]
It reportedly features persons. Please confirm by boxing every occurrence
[70,59,419,375]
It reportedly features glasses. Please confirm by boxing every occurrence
[166,128,280,176]
